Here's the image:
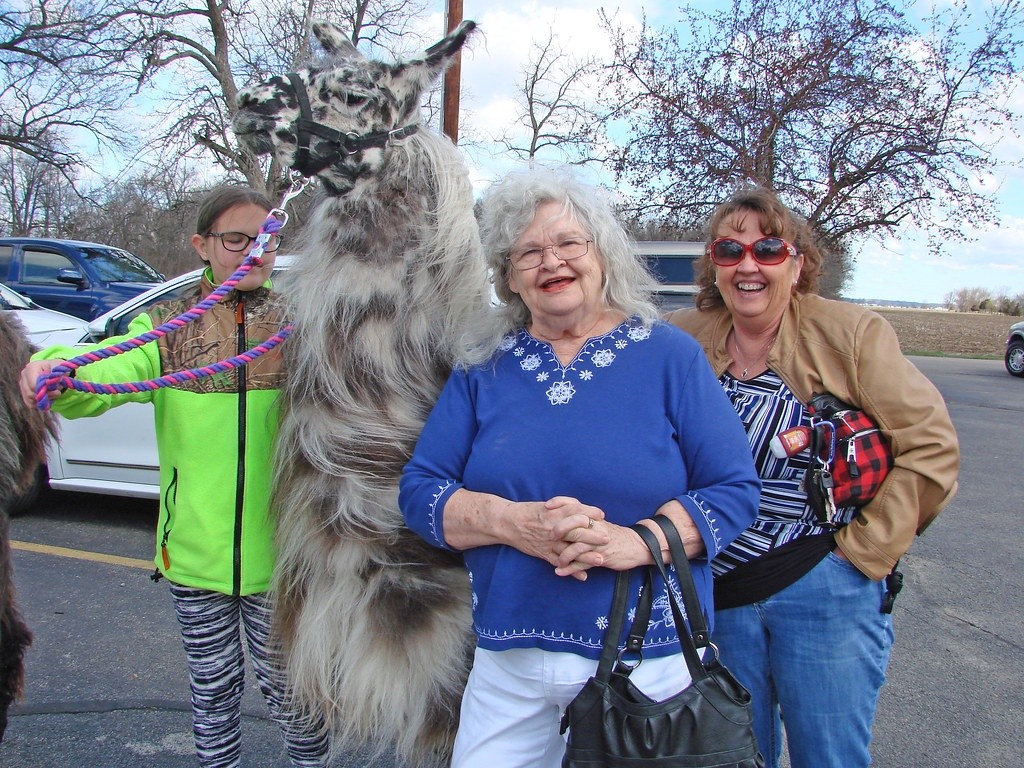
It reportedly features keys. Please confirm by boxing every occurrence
[813,469,836,522]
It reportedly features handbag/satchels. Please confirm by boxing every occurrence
[559,513,765,768]
[804,394,895,529]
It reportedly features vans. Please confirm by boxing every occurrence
[627,240,711,315]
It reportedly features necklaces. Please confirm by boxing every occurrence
[735,334,775,378]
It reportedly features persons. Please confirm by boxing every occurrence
[398,164,762,768]
[659,190,961,768]
[19,186,334,768]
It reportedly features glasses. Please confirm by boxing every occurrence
[710,236,797,266]
[206,231,284,253]
[504,237,594,271]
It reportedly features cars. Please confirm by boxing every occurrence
[0,284,94,353]
[0,255,305,533]
[0,238,171,328]
[1003,321,1024,377]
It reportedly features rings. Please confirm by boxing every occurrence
[588,518,593,529]
